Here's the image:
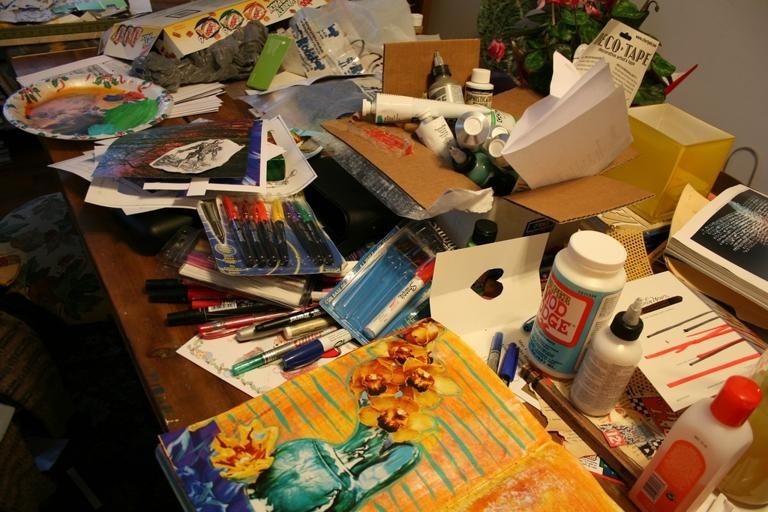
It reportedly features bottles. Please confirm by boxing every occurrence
[427,51,529,196]
[528,233,646,417]
[626,375,764,511]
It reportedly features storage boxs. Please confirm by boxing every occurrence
[317,37,653,251]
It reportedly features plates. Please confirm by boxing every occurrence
[3,74,173,141]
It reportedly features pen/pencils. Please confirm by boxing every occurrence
[362,257,436,339]
[487,332,519,387]
[145,276,355,376]
[186,194,334,291]
[524,315,536,331]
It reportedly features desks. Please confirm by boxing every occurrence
[11,47,768,511]
